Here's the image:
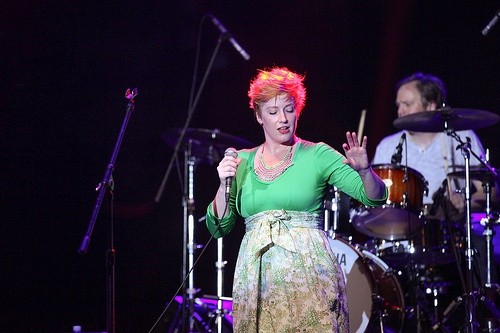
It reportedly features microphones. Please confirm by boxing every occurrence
[210,15,250,60]
[391,134,405,165]
[224,148,238,202]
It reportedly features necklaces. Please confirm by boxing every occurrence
[254,139,294,181]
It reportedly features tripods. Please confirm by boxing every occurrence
[164,124,500,333]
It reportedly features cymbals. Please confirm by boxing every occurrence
[447,164,500,185]
[177,129,255,149]
[393,108,500,130]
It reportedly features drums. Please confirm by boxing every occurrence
[426,217,466,252]
[412,266,449,299]
[325,233,404,333]
[349,163,430,241]
[374,239,416,257]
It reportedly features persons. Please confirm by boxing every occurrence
[205,66,388,333]
[370,73,496,286]
